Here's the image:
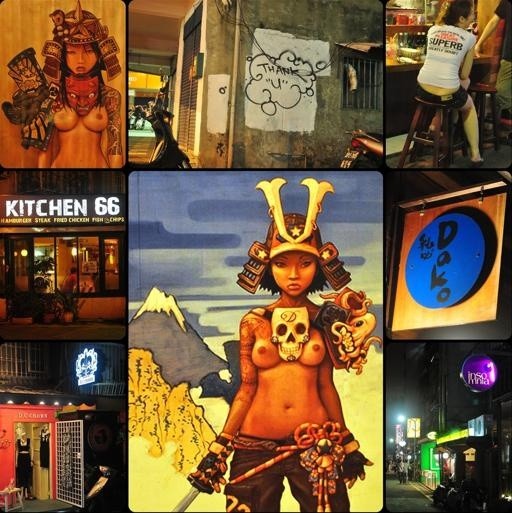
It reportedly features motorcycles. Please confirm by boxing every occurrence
[339,129,384,170]
[133,74,193,168]
[86,465,113,513]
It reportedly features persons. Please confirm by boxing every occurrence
[37,0,123,168]
[14,432,35,500]
[388,459,411,485]
[187,176,375,513]
[62,266,79,294]
[412,0,512,163]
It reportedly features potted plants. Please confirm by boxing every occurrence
[38,288,81,325]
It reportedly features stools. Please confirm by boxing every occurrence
[0,487,23,512]
[395,82,500,166]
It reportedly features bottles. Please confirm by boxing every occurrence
[397,31,427,57]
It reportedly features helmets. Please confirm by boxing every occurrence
[40,10,121,80]
[236,213,351,294]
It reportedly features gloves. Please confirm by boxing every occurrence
[338,433,370,482]
[198,432,236,487]
